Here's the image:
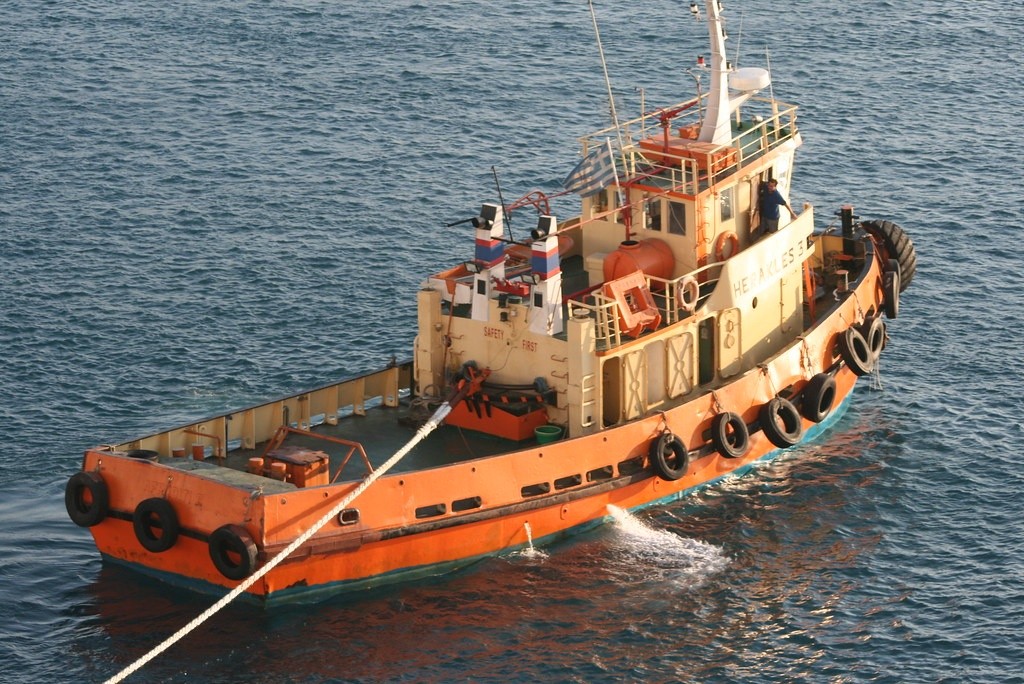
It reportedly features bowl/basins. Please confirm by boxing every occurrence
[533,425,562,444]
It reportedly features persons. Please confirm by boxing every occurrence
[758,178,798,238]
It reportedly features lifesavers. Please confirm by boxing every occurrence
[716,230,739,262]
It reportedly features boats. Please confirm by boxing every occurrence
[60,0,920,618]
